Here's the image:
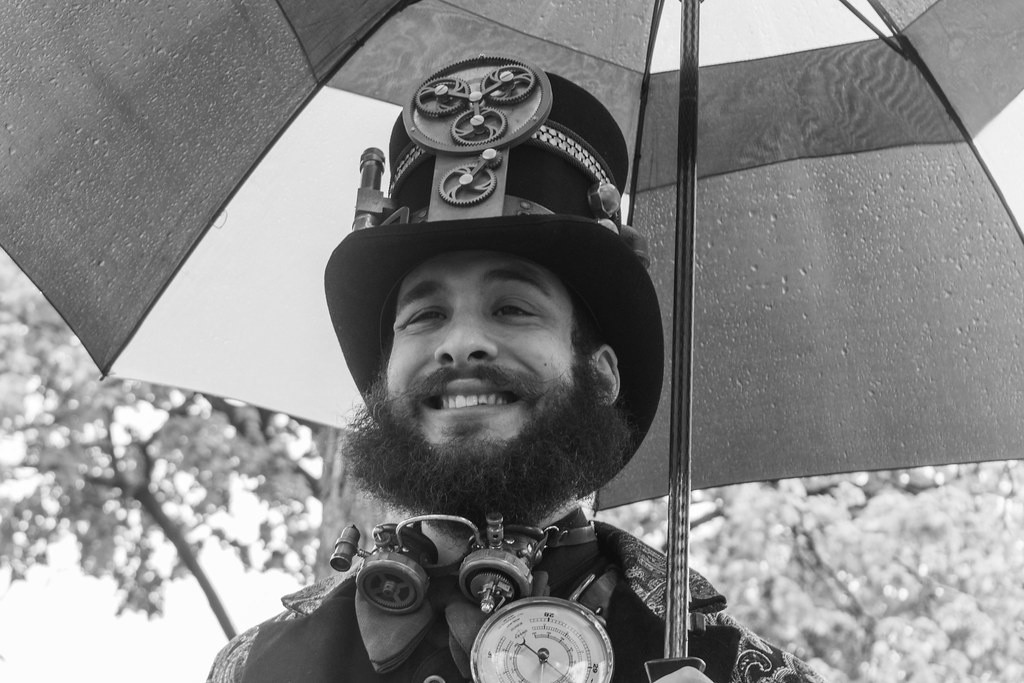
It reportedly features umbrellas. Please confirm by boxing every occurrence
[0,0,1024,683]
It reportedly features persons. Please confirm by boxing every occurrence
[207,55,821,683]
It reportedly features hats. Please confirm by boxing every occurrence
[324,57,664,499]
